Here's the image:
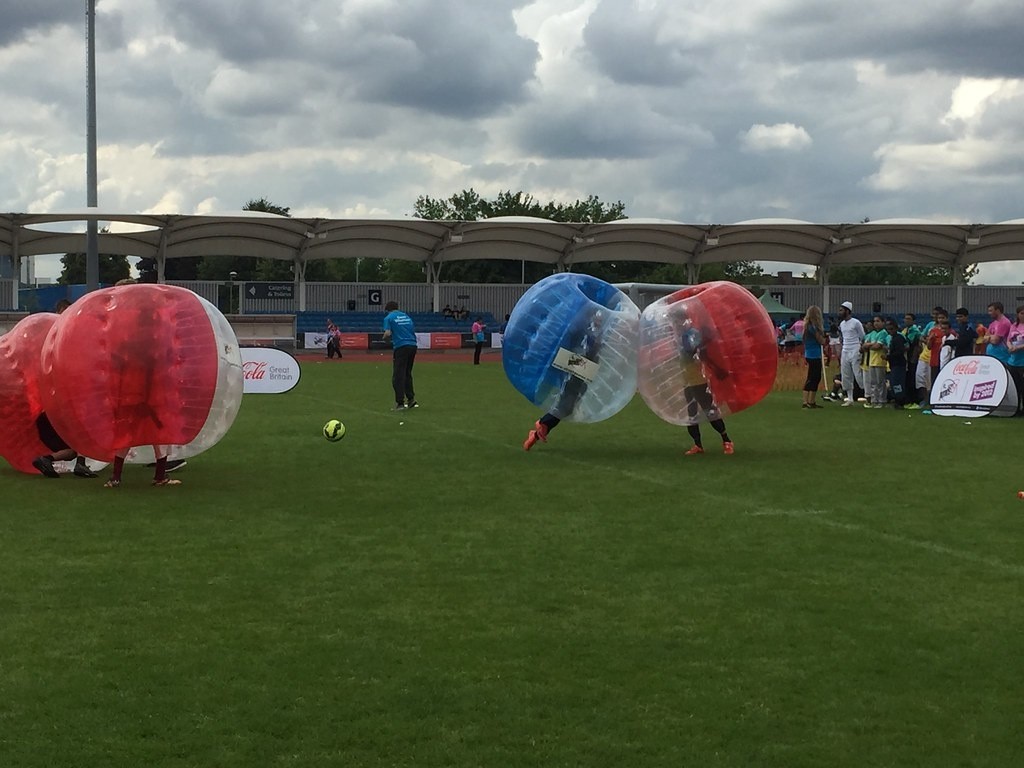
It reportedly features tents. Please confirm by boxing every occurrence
[757,292,806,323]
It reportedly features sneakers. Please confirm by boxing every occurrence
[723,440,735,455]
[74,463,100,478]
[524,430,538,450]
[535,419,548,443]
[33,456,59,479]
[152,478,181,486]
[103,479,119,487]
[685,445,704,455]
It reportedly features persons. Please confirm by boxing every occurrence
[34,299,98,478]
[673,311,734,455]
[382,301,417,410]
[105,279,185,487]
[443,305,469,322]
[772,300,1024,416]
[525,308,605,450]
[499,314,510,334]
[472,316,487,364]
[325,319,344,359]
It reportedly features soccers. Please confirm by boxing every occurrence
[323,419,346,442]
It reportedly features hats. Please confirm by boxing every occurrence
[841,301,852,311]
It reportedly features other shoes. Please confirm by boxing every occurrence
[396,404,405,410]
[165,459,187,472]
[408,400,416,407]
[802,403,823,409]
[823,392,923,409]
